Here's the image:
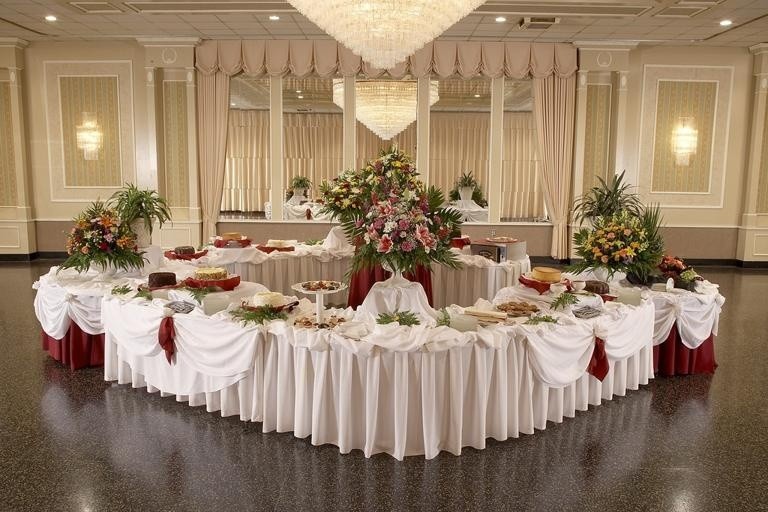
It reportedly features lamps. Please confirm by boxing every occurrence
[289,0,488,71]
[74,111,104,161]
[329,72,445,140]
[670,116,700,167]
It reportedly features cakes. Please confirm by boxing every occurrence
[193,267,228,280]
[222,231,241,240]
[252,291,284,307]
[147,272,176,287]
[532,266,562,284]
[584,280,609,294]
[175,246,195,254]
[265,239,285,248]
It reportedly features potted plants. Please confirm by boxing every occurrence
[107,181,173,248]
[448,171,488,209]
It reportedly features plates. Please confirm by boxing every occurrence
[483,236,519,244]
[496,305,541,316]
[570,290,587,294]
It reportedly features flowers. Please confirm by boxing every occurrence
[318,143,472,282]
[64,196,151,273]
[563,168,648,264]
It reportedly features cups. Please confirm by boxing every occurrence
[571,281,586,290]
[549,283,567,294]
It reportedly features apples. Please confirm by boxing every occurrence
[659,263,668,272]
